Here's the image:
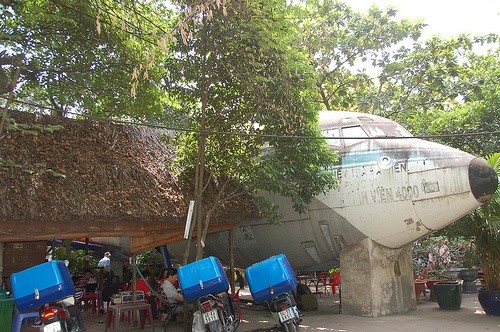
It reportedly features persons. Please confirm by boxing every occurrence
[98,252,111,270]
[129,268,185,323]
[85,270,97,285]
[429,235,451,269]
[236,272,244,290]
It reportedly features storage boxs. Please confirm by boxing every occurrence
[178,255,228,303]
[245,253,299,302]
[11,260,76,311]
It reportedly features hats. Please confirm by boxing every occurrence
[104,252,111,256]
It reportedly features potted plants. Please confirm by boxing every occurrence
[414,152,500,316]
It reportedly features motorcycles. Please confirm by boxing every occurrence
[265,293,303,332]
[197,294,238,332]
[39,304,84,332]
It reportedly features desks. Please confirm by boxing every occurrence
[105,301,152,332]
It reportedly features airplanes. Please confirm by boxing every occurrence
[0,110,496,280]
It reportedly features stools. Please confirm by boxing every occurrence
[12,312,39,332]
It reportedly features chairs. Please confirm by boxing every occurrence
[300,272,341,296]
